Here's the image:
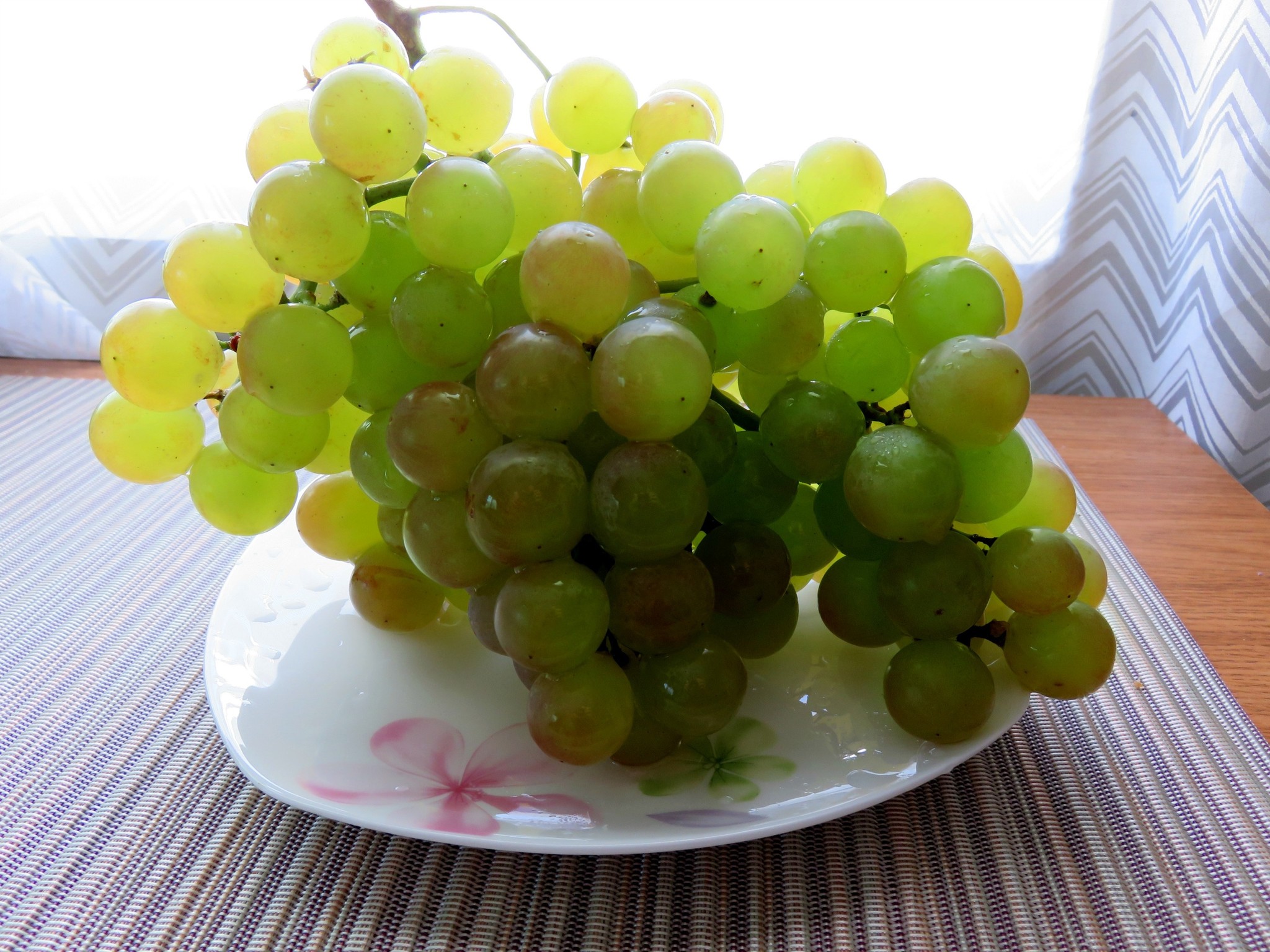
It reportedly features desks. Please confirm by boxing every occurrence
[0,345,1270,952]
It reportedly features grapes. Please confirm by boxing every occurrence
[86,18,1121,764]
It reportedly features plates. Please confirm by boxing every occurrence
[203,517,1026,856]
